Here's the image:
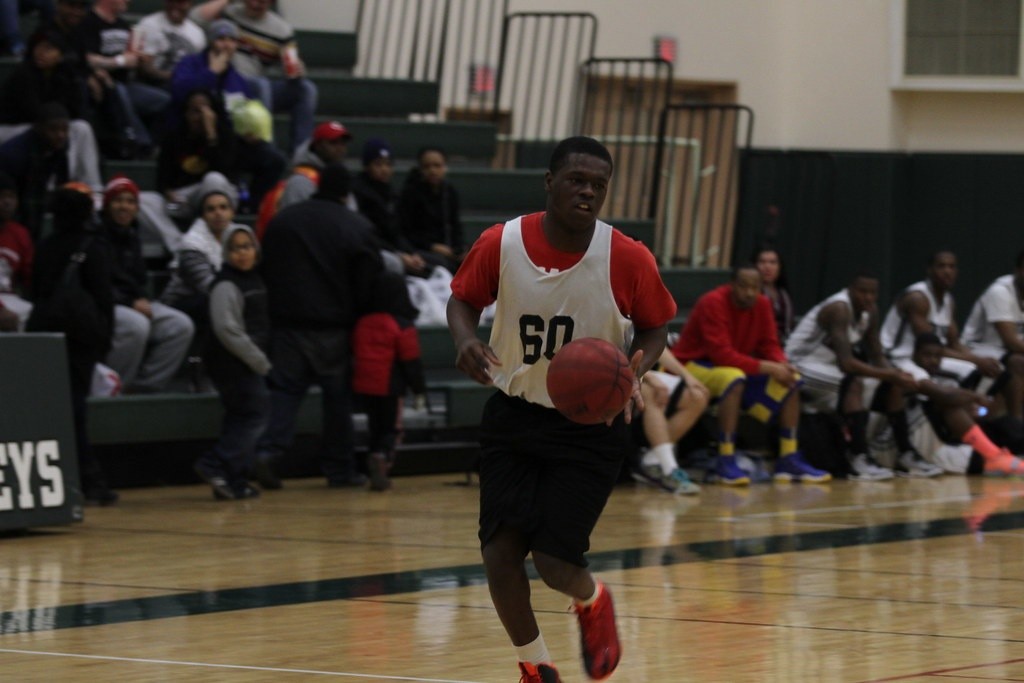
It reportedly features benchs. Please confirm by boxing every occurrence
[0,0,778,489]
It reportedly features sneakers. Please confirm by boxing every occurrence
[705,458,752,486]
[896,450,942,478]
[632,455,701,496]
[774,454,834,484]
[847,454,894,482]
[984,449,1023,477]
[516,661,565,683]
[575,582,622,681]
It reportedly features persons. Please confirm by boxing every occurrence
[627,242,1024,497]
[0,0,496,501]
[446,137,676,683]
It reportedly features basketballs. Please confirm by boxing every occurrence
[546,337,634,424]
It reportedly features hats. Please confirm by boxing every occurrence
[313,119,353,142]
[106,177,138,201]
[204,20,240,41]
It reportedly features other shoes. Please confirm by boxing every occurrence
[252,463,284,488]
[198,457,260,499]
[368,452,391,487]
[326,469,370,487]
